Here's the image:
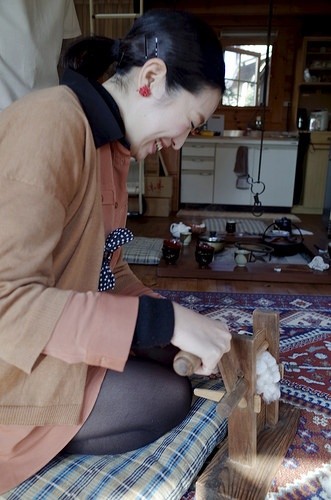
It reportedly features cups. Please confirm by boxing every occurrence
[225,221,236,233]
[194,244,214,269]
[161,240,181,265]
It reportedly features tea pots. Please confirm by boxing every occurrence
[169,221,191,238]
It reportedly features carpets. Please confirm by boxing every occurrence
[149,290,331,500]
[156,223,331,284]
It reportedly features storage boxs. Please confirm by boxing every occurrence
[145,176,174,217]
[310,109,328,132]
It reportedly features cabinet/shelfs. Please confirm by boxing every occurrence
[127,132,331,218]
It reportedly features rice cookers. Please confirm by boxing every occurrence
[308,108,328,131]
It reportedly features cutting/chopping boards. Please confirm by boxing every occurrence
[207,115,224,136]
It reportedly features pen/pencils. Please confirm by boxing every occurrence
[289,365,331,369]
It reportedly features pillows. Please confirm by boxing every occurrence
[0,377,230,500]
[122,237,163,264]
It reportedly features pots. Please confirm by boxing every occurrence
[198,231,224,253]
[262,222,304,257]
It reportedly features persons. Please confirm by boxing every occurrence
[0,7,232,494]
